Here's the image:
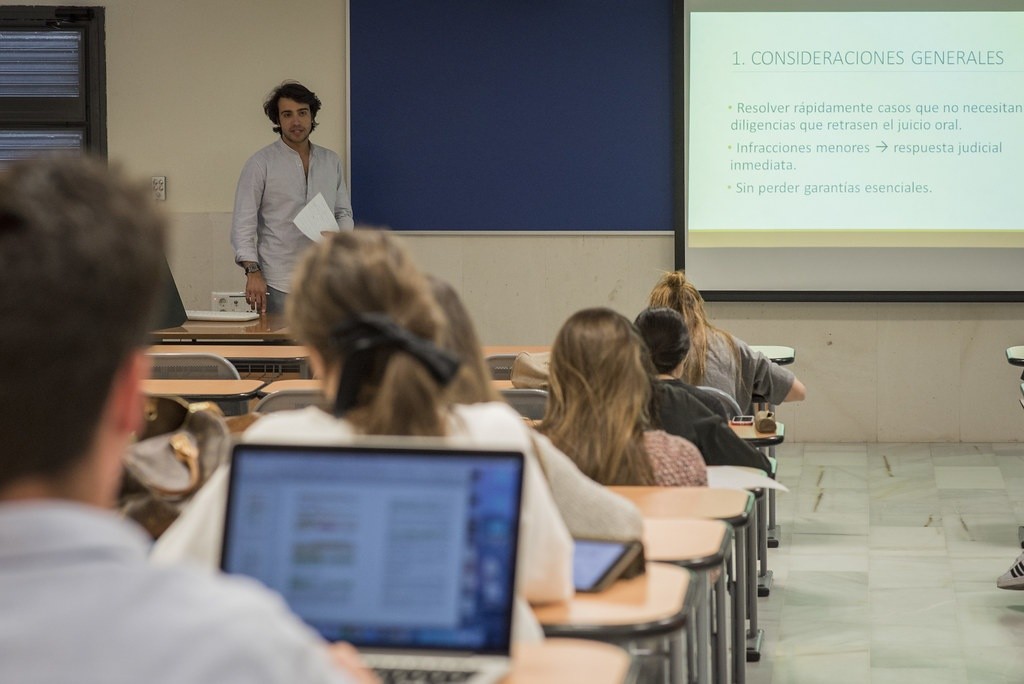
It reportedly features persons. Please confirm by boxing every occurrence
[524,308,709,486]
[0,143,385,684]
[231,81,354,320]
[634,304,773,477]
[647,272,807,419]
[151,229,642,647]
[996,526,1024,591]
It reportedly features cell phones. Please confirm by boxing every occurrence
[732,415,754,425]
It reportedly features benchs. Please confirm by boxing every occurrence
[141,377,267,418]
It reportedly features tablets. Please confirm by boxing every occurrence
[569,536,641,593]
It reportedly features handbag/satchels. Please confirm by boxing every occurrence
[123,395,231,536]
[510,351,553,392]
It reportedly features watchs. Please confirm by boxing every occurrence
[245,263,262,274]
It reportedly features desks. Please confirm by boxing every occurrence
[502,637,640,684]
[477,342,795,549]
[1006,345,1024,366]
[596,486,758,684]
[728,422,784,598]
[145,345,315,382]
[641,516,733,684]
[258,377,516,401]
[532,562,706,684]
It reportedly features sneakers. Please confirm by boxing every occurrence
[997,551,1024,590]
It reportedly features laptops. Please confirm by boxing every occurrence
[219,443,526,684]
